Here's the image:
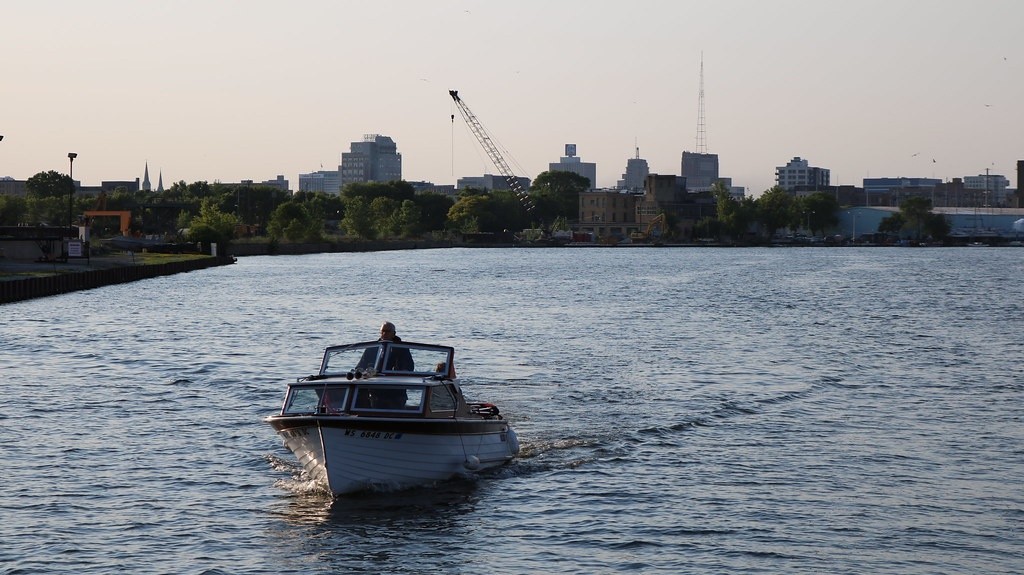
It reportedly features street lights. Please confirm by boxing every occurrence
[67,152,77,238]
[634,195,642,233]
[847,210,862,243]
[802,210,815,237]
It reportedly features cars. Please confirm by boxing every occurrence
[771,231,899,243]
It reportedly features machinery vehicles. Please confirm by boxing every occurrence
[630,213,667,246]
[449,89,575,245]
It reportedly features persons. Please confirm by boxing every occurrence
[356,322,416,410]
[426,363,457,410]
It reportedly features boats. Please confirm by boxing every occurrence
[265,340,522,502]
